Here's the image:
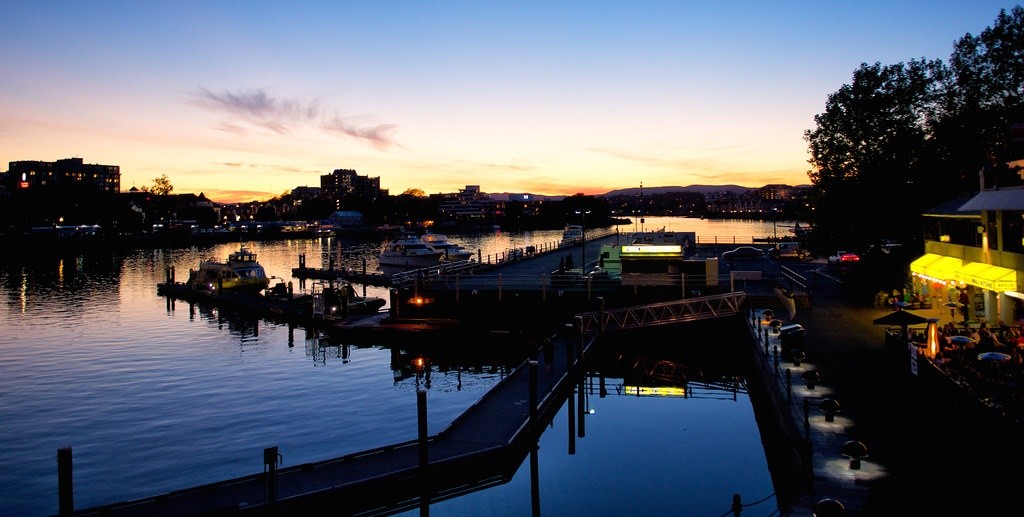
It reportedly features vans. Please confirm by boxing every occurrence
[767,242,811,260]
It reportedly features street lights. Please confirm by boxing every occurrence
[612,209,623,246]
[575,208,591,276]
[631,209,640,232]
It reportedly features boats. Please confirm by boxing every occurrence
[264,282,386,316]
[561,225,586,244]
[420,229,475,262]
[187,250,272,293]
[379,226,445,267]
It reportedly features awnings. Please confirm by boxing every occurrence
[910,253,1017,292]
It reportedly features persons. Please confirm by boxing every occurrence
[908,322,1024,406]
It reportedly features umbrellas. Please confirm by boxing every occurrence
[873,310,927,334]
[949,335,976,349]
[978,352,1010,369]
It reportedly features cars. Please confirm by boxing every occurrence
[826,251,859,265]
[722,246,765,261]
